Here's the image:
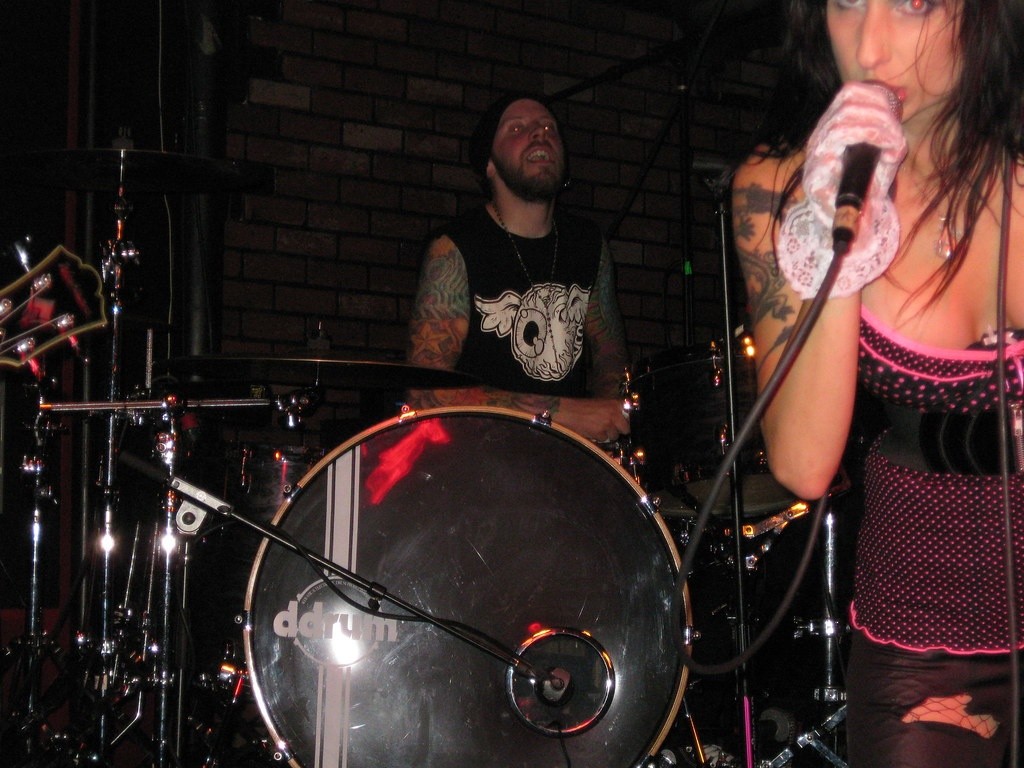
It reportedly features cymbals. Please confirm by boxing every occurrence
[15,147,246,199]
[177,352,485,388]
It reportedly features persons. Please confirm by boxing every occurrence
[731,0,1024,768]
[402,89,633,443]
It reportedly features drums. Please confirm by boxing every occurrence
[618,346,848,517]
[234,405,702,768]
[226,439,330,547]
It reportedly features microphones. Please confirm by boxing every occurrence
[830,81,905,256]
[519,663,577,707]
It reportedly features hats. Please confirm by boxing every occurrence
[470,90,559,171]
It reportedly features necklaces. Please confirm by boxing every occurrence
[903,161,962,260]
[490,199,559,360]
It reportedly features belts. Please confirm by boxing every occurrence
[846,381,1024,477]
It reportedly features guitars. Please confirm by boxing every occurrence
[0,243,109,370]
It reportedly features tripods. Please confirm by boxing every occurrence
[0,156,287,768]
